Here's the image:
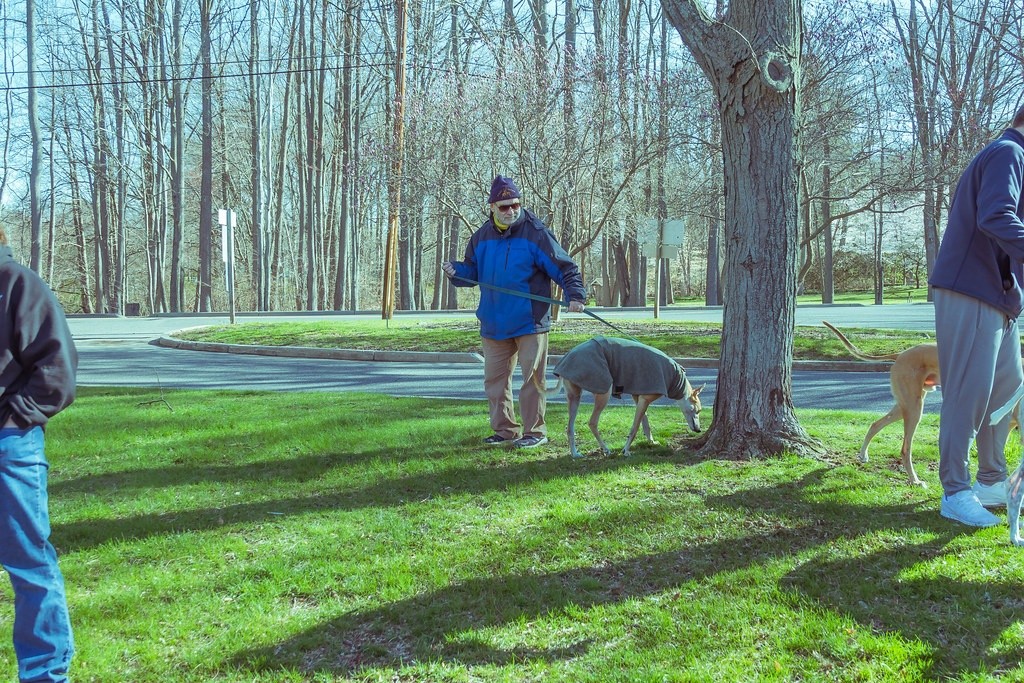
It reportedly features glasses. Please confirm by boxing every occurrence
[494,202,521,212]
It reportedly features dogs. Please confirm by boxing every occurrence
[1002,462,1024,546]
[531,337,707,457]
[820,319,1024,489]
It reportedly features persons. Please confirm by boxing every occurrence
[0,225,80,683]
[930,103,1024,525]
[443,174,587,449]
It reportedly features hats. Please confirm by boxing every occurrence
[488,175,520,202]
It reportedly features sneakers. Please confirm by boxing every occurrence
[512,436,548,448]
[483,434,522,444]
[973,480,1024,508]
[941,490,1001,527]
